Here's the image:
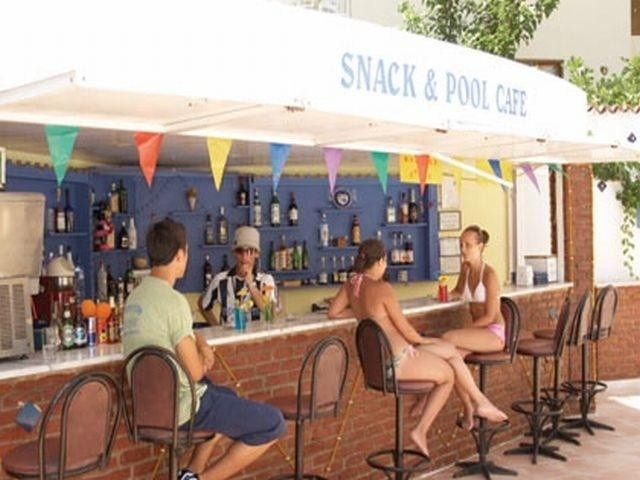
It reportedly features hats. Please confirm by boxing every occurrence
[232,226,261,253]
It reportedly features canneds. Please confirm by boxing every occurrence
[85,316,97,347]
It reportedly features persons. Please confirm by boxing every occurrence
[408,224,506,432]
[197,225,276,327]
[122,216,286,480]
[326,238,508,458]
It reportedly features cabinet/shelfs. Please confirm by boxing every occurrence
[253,178,441,286]
[91,174,251,301]
[6,159,90,301]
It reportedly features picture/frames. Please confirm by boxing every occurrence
[437,210,462,231]
[439,236,460,256]
[440,257,461,274]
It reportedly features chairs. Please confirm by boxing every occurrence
[122,346,213,478]
[561,285,618,434]
[452,298,518,479]
[355,317,435,479]
[526,292,591,448]
[4,371,121,479]
[266,337,347,479]
[504,299,569,465]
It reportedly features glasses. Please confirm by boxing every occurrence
[234,248,258,257]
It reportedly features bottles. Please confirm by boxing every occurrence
[46,178,138,349]
[203,177,427,288]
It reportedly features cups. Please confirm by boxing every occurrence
[265,304,272,321]
[234,307,246,330]
[41,326,61,359]
[439,284,447,302]
